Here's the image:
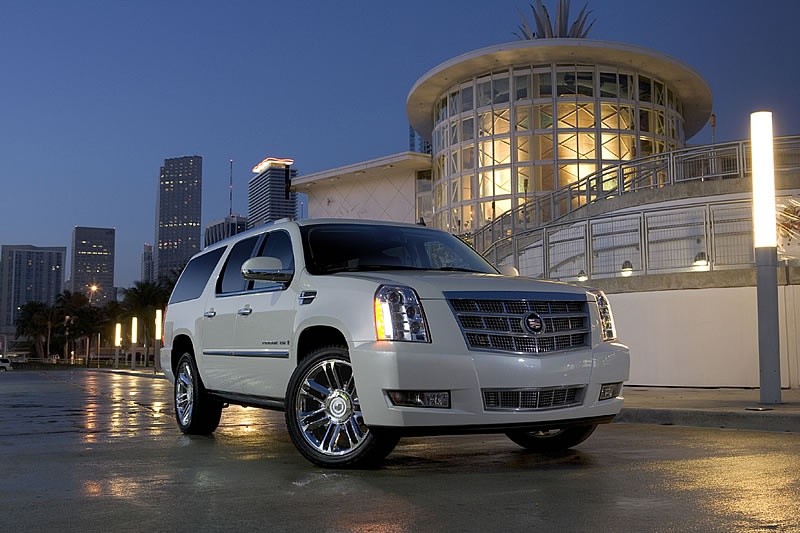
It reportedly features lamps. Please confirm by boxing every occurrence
[577,249,713,282]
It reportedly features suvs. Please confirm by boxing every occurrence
[0,358,14,372]
[158,214,631,471]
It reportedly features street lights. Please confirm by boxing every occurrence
[114,322,121,368]
[86,284,100,368]
[746,106,783,406]
[129,316,138,369]
[153,308,163,372]
